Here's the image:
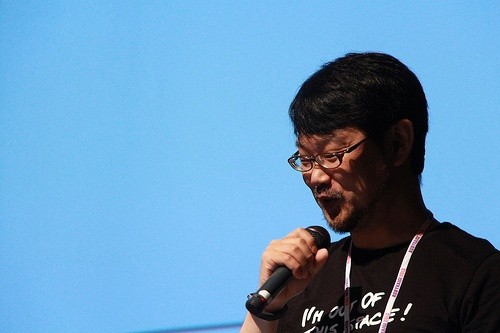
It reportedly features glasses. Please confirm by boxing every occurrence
[287,136,370,172]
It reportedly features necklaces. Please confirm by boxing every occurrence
[342,211,434,333]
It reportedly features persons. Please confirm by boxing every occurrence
[236,50,500,333]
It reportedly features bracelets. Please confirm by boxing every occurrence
[246,294,289,322]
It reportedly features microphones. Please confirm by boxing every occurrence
[246,226,331,315]
